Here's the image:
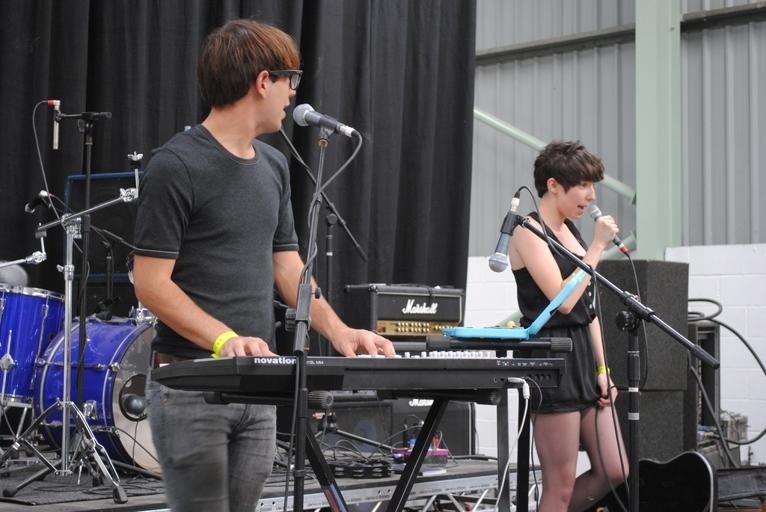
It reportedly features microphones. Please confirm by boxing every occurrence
[489,198,520,272]
[588,203,629,256]
[48,99,60,149]
[293,103,356,138]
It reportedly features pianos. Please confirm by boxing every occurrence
[151,350,565,393]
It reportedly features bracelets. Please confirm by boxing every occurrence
[211,332,238,359]
[597,366,610,374]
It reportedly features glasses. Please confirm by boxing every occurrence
[266,69,303,89]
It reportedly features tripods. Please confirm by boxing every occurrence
[0,129,164,505]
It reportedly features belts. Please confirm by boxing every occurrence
[148,350,195,370]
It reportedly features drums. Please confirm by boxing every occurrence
[33,313,160,475]
[0,283,64,408]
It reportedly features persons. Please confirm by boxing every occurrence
[509,140,630,512]
[132,18,396,512]
